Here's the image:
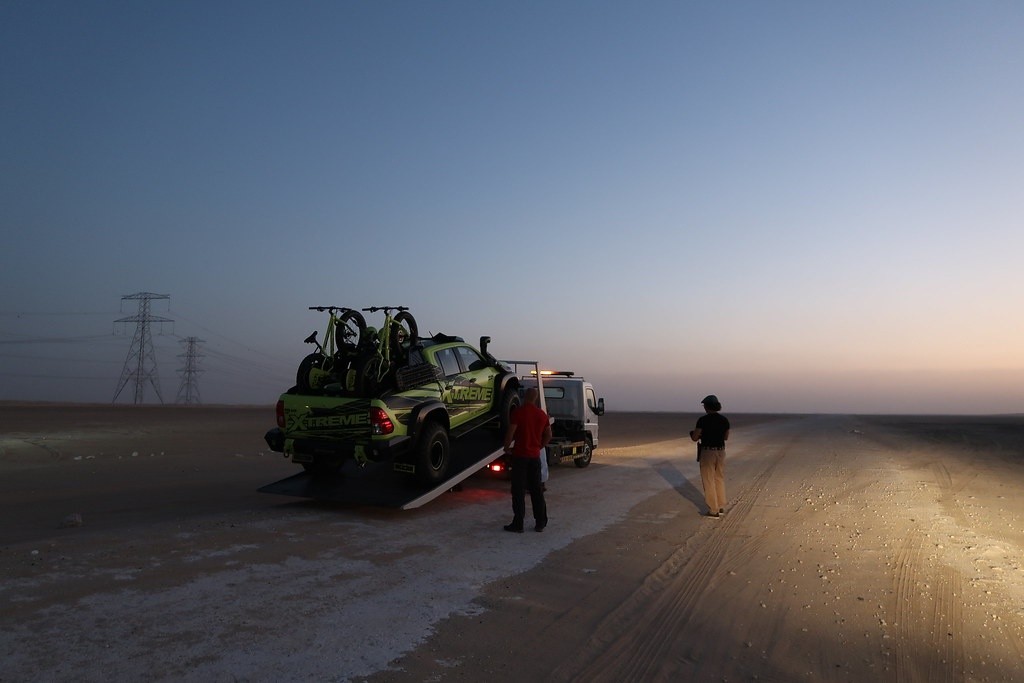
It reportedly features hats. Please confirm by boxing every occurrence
[701,395,719,404]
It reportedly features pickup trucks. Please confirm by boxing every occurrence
[264,331,524,488]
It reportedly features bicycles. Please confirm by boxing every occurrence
[345,305,419,398]
[296,305,366,398]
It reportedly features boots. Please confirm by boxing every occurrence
[531,501,547,533]
[503,496,526,533]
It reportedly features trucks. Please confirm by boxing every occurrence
[255,360,604,511]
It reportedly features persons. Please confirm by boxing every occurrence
[690,395,729,519]
[503,387,553,533]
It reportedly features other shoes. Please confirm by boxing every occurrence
[719,508,724,514]
[702,512,721,519]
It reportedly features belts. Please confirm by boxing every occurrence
[701,446,727,450]
